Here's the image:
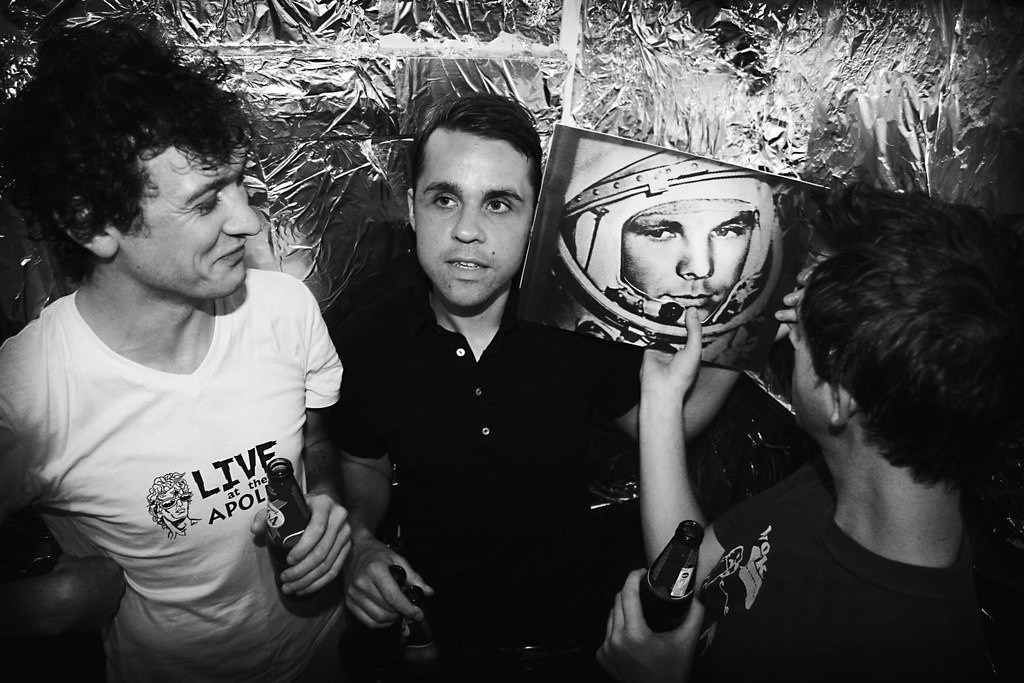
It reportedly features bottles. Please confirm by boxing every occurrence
[266,458,332,618]
[640,521,704,632]
[337,566,406,658]
[403,586,441,679]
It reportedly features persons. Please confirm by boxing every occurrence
[558,137,783,369]
[598,181,1024,683]
[329,93,816,683]
[0,39,352,683]
